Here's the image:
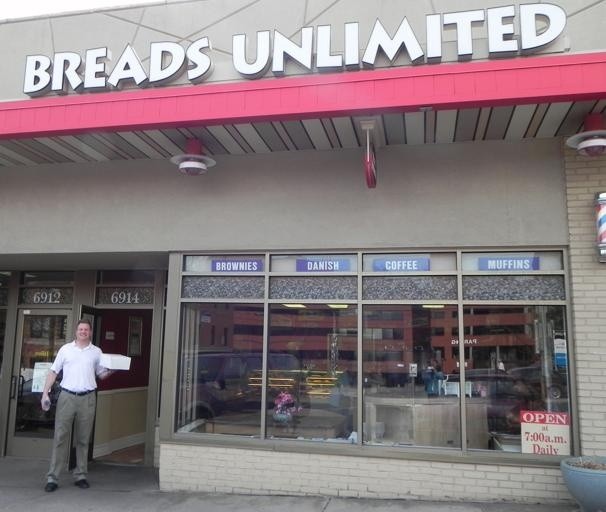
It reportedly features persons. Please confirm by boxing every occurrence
[38,317,118,493]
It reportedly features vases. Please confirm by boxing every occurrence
[560,455,606,512]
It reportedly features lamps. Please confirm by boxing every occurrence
[169,138,217,176]
[566,112,606,156]
[593,192,606,263]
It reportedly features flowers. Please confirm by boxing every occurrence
[273,391,304,417]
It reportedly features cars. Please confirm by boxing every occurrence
[16,372,61,432]
[441,367,566,435]
[197,352,311,418]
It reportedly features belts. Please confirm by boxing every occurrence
[59,386,96,397]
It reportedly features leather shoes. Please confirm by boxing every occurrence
[45,481,58,492]
[74,478,90,489]
